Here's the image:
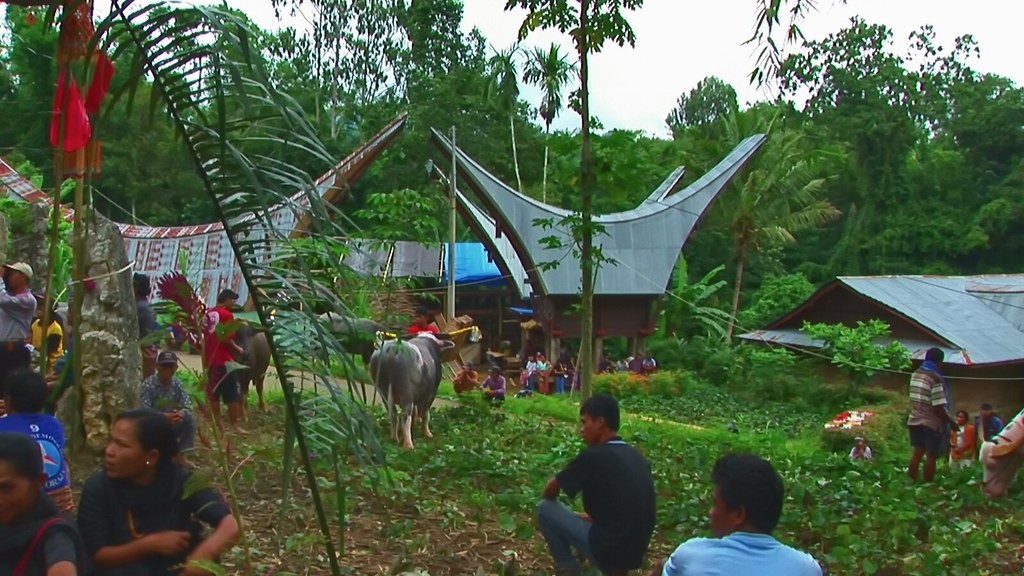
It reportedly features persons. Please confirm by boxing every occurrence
[486,334,513,371]
[907,347,961,483]
[949,409,977,474]
[479,365,506,410]
[662,453,823,576]
[517,341,657,399]
[453,360,481,397]
[536,389,657,576]
[165,313,202,354]
[849,436,872,462]
[131,272,161,383]
[0,366,76,520]
[202,290,252,436]
[974,401,1004,459]
[75,409,238,576]
[0,258,76,414]
[0,430,87,576]
[138,350,199,471]
[406,308,441,422]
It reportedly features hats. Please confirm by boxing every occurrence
[158,350,177,365]
[2,261,34,279]
[463,361,473,369]
[488,366,501,373]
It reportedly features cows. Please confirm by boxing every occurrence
[368,323,485,453]
[310,311,397,376]
[229,317,272,424]
